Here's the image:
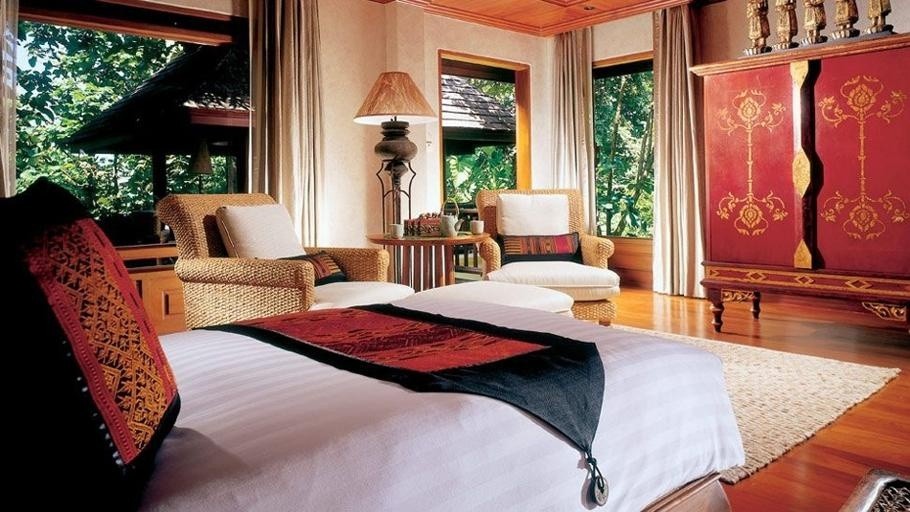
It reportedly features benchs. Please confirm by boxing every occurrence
[426,278,577,315]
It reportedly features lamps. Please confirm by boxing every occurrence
[186,141,213,191]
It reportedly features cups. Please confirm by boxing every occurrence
[388,223,405,241]
[469,220,485,235]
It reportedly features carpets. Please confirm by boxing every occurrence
[612,319,901,489]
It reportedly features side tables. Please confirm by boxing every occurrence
[366,229,491,292]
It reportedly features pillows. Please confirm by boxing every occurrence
[210,202,348,286]
[0,176,180,511]
[496,192,584,269]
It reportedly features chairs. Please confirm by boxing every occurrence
[154,189,392,327]
[472,188,621,327]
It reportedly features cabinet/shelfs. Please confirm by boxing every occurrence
[681,36,910,338]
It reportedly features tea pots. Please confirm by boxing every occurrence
[438,197,466,237]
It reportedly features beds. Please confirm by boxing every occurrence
[145,292,740,512]
